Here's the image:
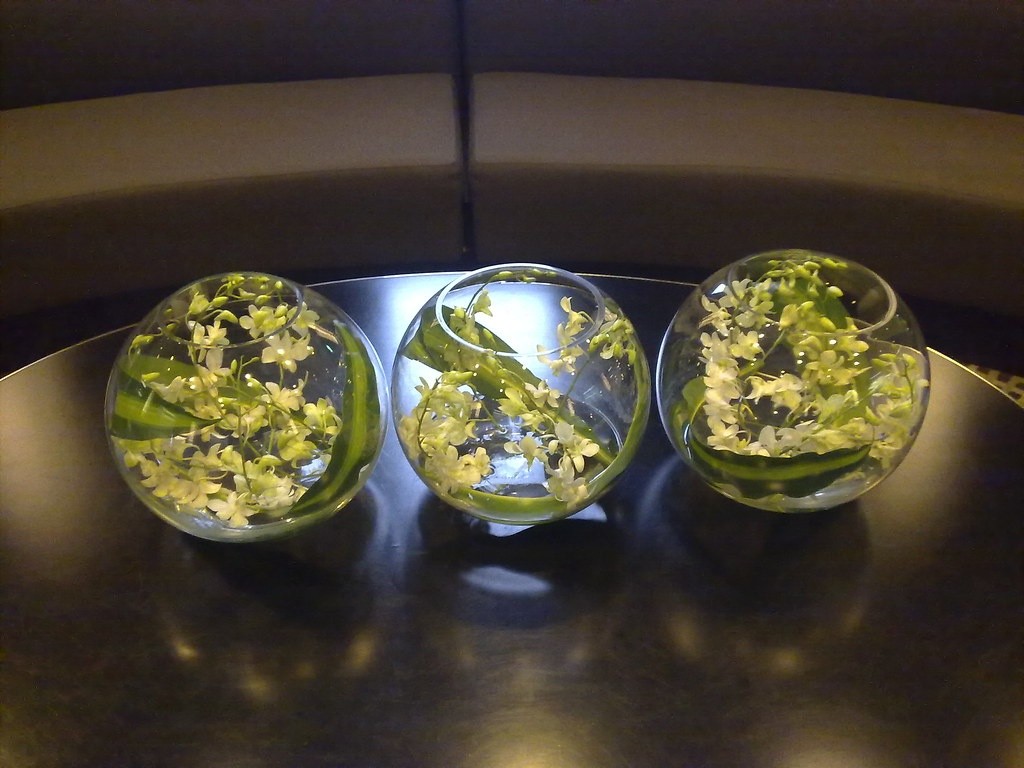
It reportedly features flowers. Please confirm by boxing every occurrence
[398,267,648,519]
[108,275,382,529]
[672,257,921,498]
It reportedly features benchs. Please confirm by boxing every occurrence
[0,0,1023,325]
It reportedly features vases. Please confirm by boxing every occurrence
[103,272,393,543]
[389,262,652,527]
[655,250,932,514]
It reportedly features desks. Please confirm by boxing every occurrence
[0,269,1023,768]
